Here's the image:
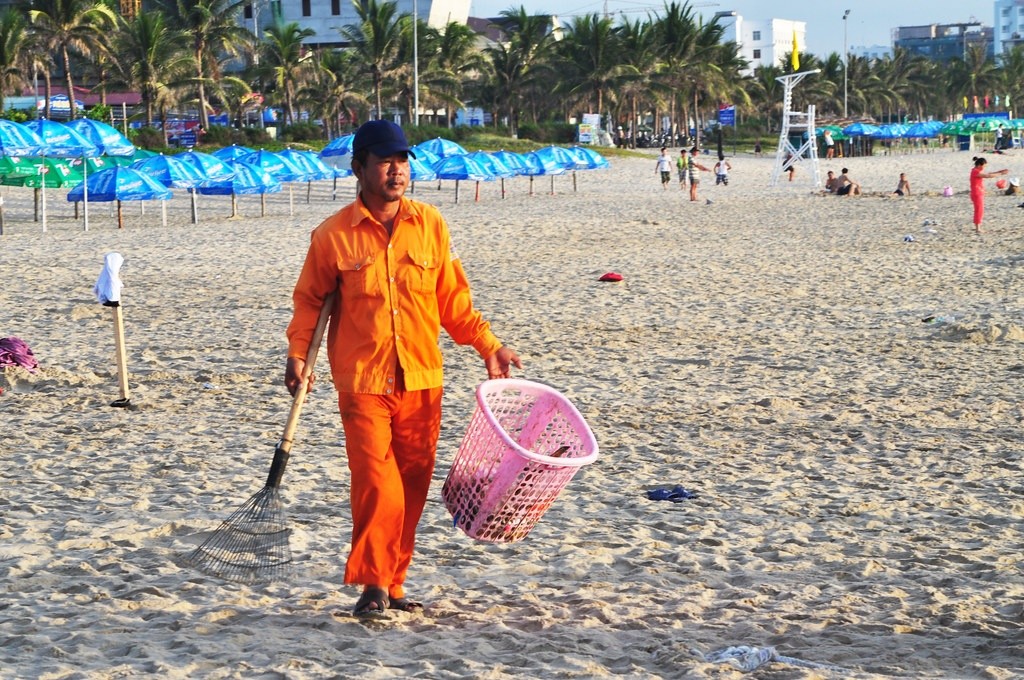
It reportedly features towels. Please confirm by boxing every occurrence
[92,252,125,306]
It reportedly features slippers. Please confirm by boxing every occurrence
[353,588,390,617]
[389,598,423,612]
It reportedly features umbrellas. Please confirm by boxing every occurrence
[0,115,609,231]
[804,117,1024,156]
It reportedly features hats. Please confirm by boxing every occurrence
[353,120,416,161]
[1010,177,1019,187]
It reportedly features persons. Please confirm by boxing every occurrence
[824,130,835,160]
[655,148,673,190]
[688,146,708,203]
[782,154,796,181]
[754,141,761,157]
[995,124,1008,150]
[615,126,632,148]
[714,154,731,185]
[923,137,929,147]
[677,149,688,189]
[286,120,521,619]
[914,138,920,147]
[944,135,950,147]
[970,157,1009,233]
[891,173,910,196]
[825,168,861,196]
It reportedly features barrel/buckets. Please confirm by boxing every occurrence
[996,179,1007,188]
[944,186,953,196]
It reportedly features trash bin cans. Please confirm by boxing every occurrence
[441,377,598,543]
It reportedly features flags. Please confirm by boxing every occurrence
[964,95,1010,109]
[791,32,799,70]
